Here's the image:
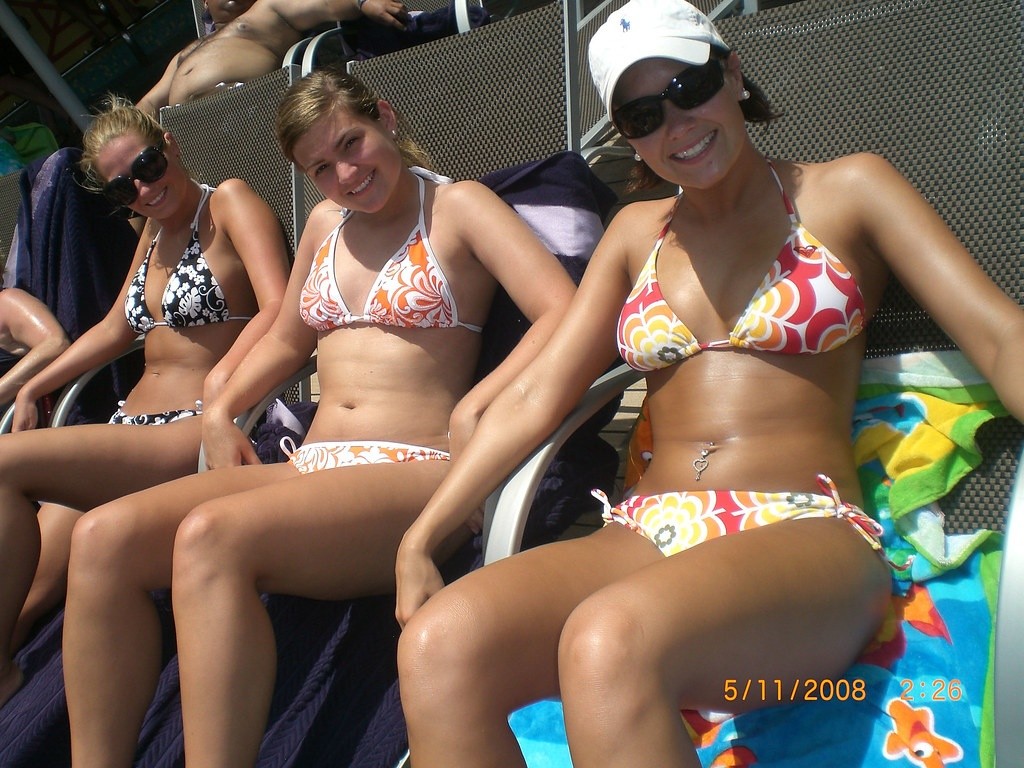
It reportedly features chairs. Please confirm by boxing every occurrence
[0,0,1024,768]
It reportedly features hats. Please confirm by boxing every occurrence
[588,0,731,122]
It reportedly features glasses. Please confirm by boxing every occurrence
[101,140,168,206]
[613,44,725,139]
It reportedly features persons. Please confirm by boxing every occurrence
[61,76,577,768]
[0,260,71,432]
[134,0,409,124]
[0,93,291,707]
[393,0,1024,768]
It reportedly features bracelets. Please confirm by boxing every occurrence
[358,0,366,11]
